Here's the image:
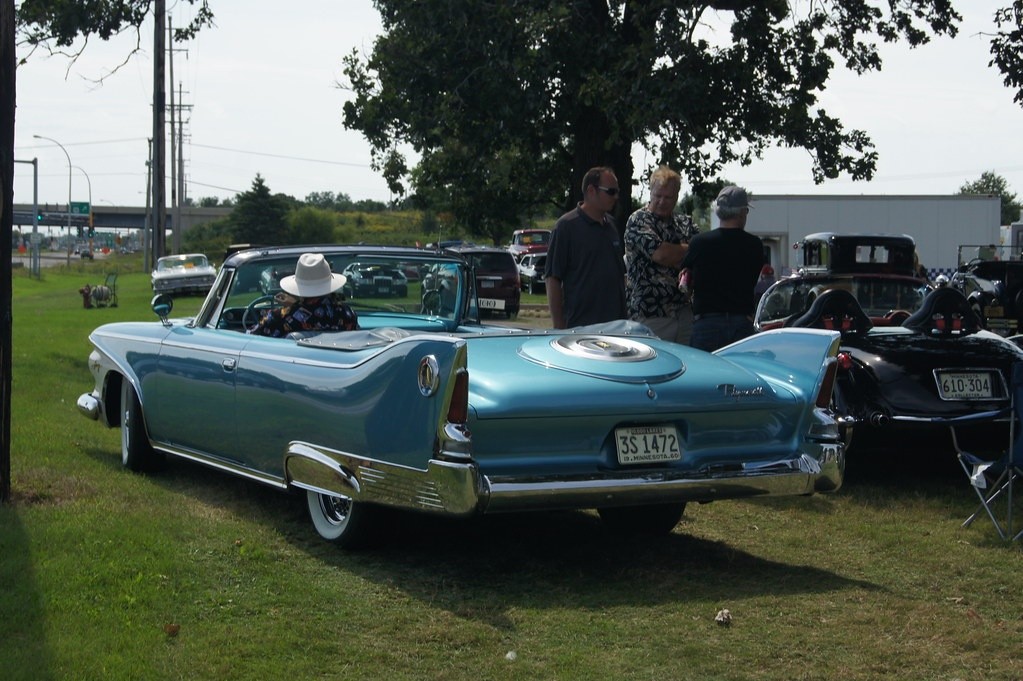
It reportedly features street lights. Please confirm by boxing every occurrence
[63,164,94,259]
[37,208,43,225]
[33,131,72,265]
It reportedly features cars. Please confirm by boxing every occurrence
[77,246,844,546]
[341,262,408,300]
[747,272,1023,496]
[259,261,290,297]
[517,253,548,295]
[150,253,219,298]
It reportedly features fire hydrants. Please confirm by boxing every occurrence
[79,283,92,308]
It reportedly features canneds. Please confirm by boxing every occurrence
[678,271,692,294]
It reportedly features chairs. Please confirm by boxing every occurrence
[790,287,873,334]
[901,286,982,336]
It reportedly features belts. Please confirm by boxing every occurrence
[691,312,732,322]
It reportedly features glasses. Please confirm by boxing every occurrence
[594,185,620,195]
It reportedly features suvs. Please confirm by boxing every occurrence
[508,229,552,264]
[222,242,272,294]
[936,243,1023,340]
[782,232,919,315]
[420,246,521,320]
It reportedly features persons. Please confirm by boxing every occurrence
[683,184,766,353]
[246,251,361,344]
[755,267,776,300]
[543,168,630,331]
[625,166,698,346]
[913,237,1001,295]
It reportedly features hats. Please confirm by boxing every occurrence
[716,186,755,209]
[279,252,346,297]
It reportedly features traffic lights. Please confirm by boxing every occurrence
[87,226,93,238]
[76,227,81,238]
